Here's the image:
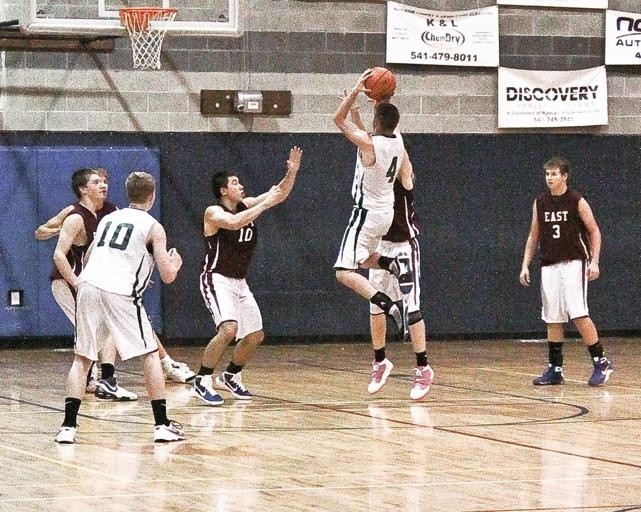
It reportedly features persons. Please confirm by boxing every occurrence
[337,88,434,401]
[56,171,187,444]
[49,168,154,401]
[333,67,415,343]
[34,168,197,392]
[519,156,614,387]
[191,145,303,406]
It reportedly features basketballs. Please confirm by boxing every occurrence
[364,68,396,100]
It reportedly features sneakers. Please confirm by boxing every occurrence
[533,366,565,385]
[410,364,434,400]
[189,381,224,406]
[367,357,393,395]
[153,423,186,441]
[86,377,138,400]
[166,363,195,383]
[54,426,75,442]
[588,357,613,385]
[216,372,251,400]
[388,299,408,343]
[390,252,412,294]
